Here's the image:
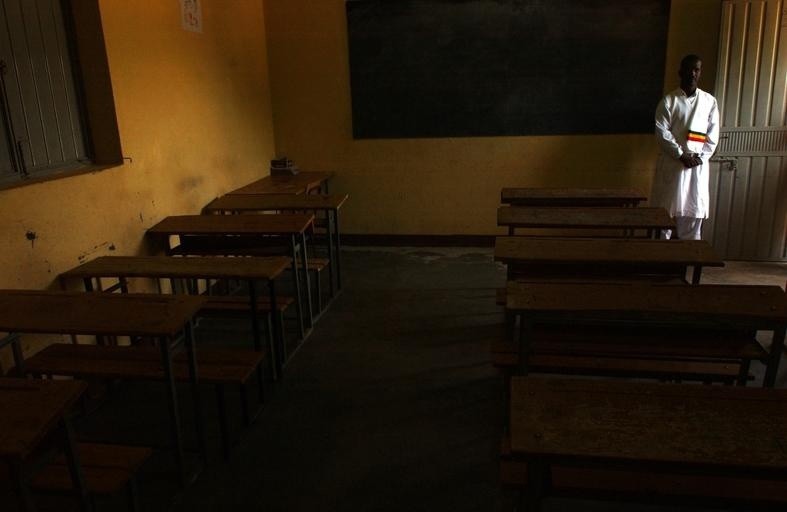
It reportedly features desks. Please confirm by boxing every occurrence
[496,187,787,511]
[1,169,347,511]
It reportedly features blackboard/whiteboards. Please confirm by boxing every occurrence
[345,0,672,140]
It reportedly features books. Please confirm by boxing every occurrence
[270,166,292,176]
[271,159,293,168]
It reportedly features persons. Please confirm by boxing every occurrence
[649,54,723,242]
